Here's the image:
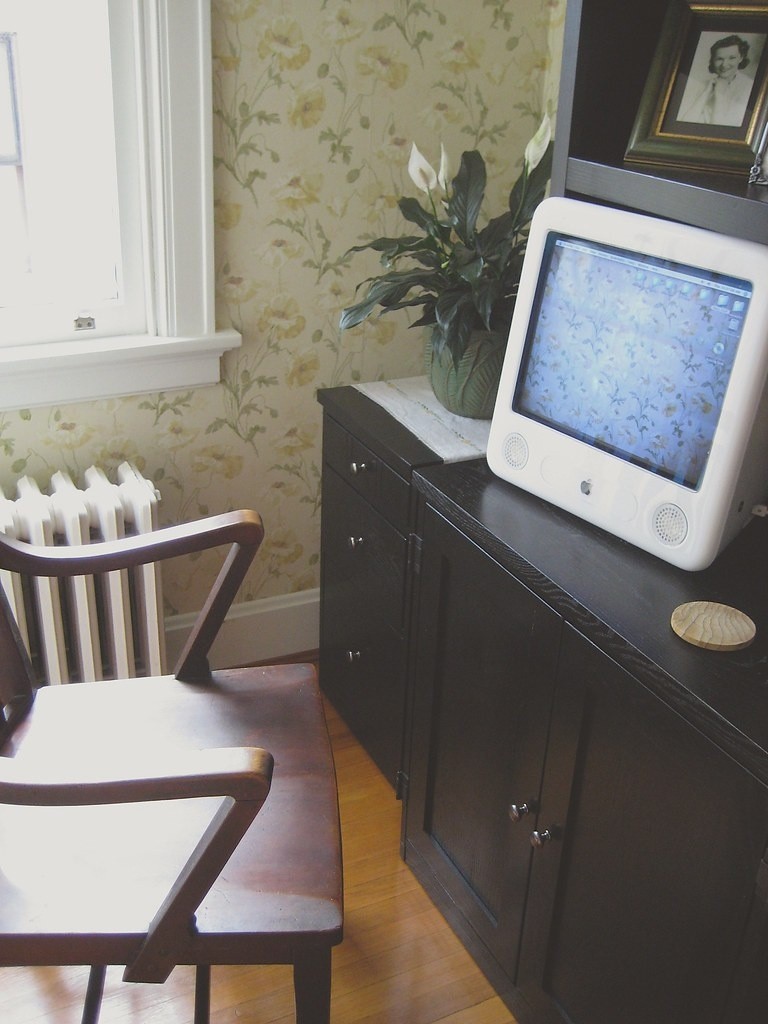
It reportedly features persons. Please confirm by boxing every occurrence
[678,34,754,127]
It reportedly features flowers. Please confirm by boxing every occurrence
[339,109,556,373]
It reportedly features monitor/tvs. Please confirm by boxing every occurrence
[486,197,768,572]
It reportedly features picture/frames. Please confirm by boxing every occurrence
[624,1,768,174]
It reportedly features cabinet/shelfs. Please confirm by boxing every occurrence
[398,459,768,1024]
[317,386,443,799]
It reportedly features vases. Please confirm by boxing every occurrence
[430,311,511,419]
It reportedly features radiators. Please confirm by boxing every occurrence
[0,461,167,686]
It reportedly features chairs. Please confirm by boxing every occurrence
[0,509,344,1024]
